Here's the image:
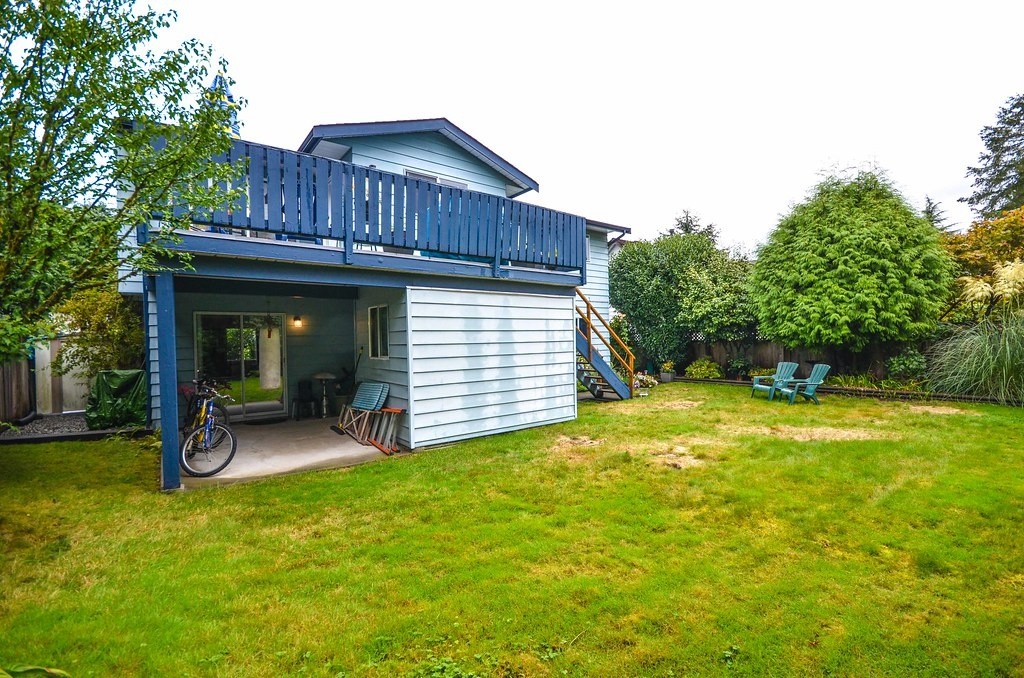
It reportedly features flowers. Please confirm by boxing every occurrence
[662,361,676,372]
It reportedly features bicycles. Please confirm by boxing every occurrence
[179,369,237,478]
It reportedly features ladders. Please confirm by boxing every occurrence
[337,402,407,457]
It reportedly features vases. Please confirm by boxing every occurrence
[661,373,674,382]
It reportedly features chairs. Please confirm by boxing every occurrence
[750,362,831,405]
[292,379,316,418]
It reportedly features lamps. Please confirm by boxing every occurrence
[293,314,303,328]
